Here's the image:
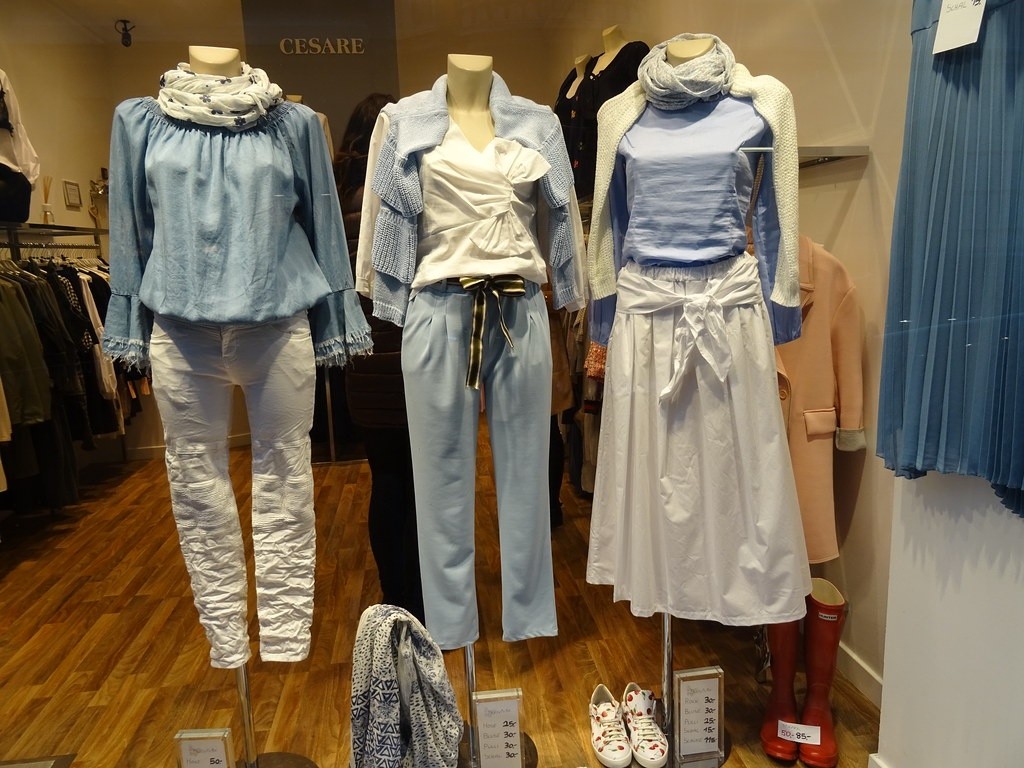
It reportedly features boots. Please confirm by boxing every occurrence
[797,578,846,768]
[761,620,799,764]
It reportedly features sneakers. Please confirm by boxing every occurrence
[623,682,671,768]
[589,684,633,768]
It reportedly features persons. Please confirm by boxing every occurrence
[331,91,427,656]
[102,46,373,668]
[550,24,811,667]
[348,54,587,653]
